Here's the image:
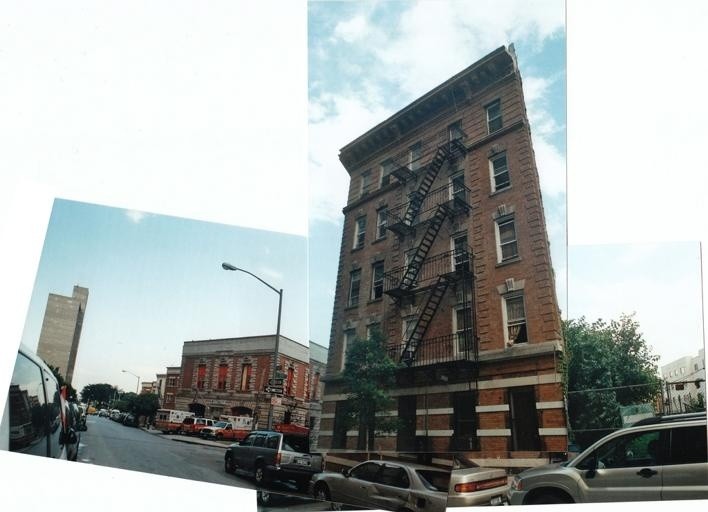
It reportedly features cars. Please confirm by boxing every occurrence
[87,404,137,428]
[256,490,320,509]
[0,343,88,462]
[306,458,451,512]
[445,453,508,507]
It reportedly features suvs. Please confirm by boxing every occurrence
[223,424,325,486]
[506,412,707,507]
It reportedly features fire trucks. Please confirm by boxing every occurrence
[154,407,254,442]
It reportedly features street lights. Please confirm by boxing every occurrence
[220,261,284,430]
[122,369,141,397]
